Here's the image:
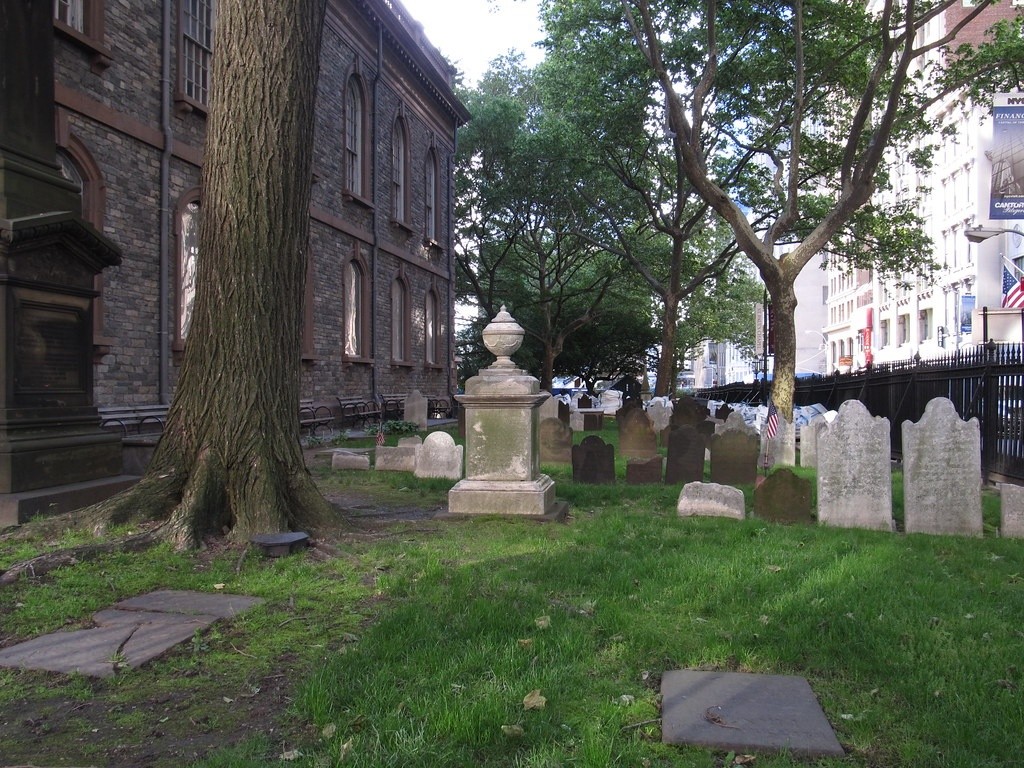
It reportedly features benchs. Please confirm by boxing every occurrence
[98,406,170,448]
[382,393,404,420]
[298,398,336,436]
[336,396,384,428]
[422,395,450,418]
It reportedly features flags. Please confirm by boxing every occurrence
[765,401,779,439]
[1001,265,1024,308]
